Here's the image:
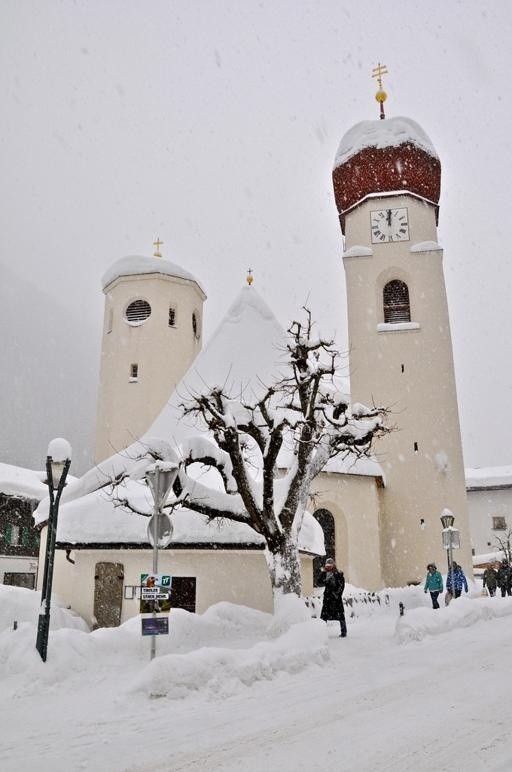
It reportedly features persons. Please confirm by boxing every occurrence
[317,557,347,637]
[423,562,443,608]
[446,562,469,600]
[498,559,512,597]
[482,563,499,597]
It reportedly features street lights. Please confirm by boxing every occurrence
[439,509,455,600]
[36,438,72,661]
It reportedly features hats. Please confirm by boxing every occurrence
[503,559,508,564]
[326,558,334,567]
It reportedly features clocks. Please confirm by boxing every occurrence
[370,207,410,244]
